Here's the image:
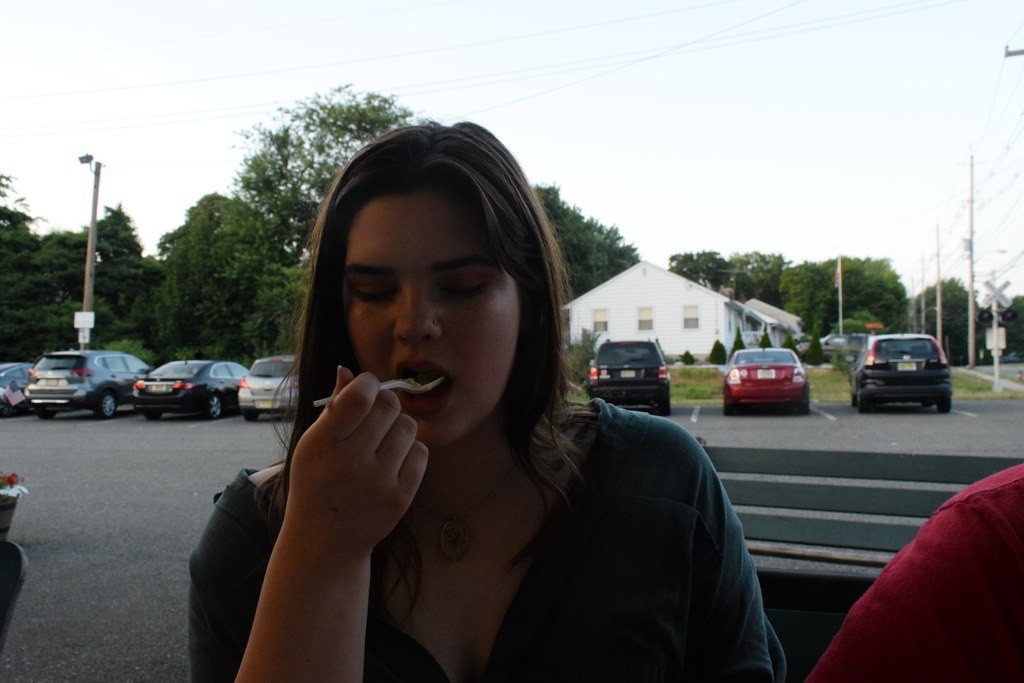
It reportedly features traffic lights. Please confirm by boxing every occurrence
[978,310,993,326]
[1002,309,1017,323]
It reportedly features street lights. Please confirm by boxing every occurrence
[79,154,101,351]
[967,250,1007,370]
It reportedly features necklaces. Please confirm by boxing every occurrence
[417,460,518,560]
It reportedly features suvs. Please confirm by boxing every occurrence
[587,338,670,416]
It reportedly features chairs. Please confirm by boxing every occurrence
[605,350,620,361]
[911,346,931,359]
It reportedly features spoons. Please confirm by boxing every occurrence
[314,378,451,408]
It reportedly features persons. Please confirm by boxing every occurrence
[802,464,1023,683]
[183,122,789,683]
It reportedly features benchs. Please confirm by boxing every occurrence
[704,443,1024,683]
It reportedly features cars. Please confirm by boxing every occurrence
[845,334,952,414]
[24,350,154,419]
[722,348,809,415]
[132,360,249,420]
[796,333,870,364]
[0,363,37,417]
[237,356,300,422]
